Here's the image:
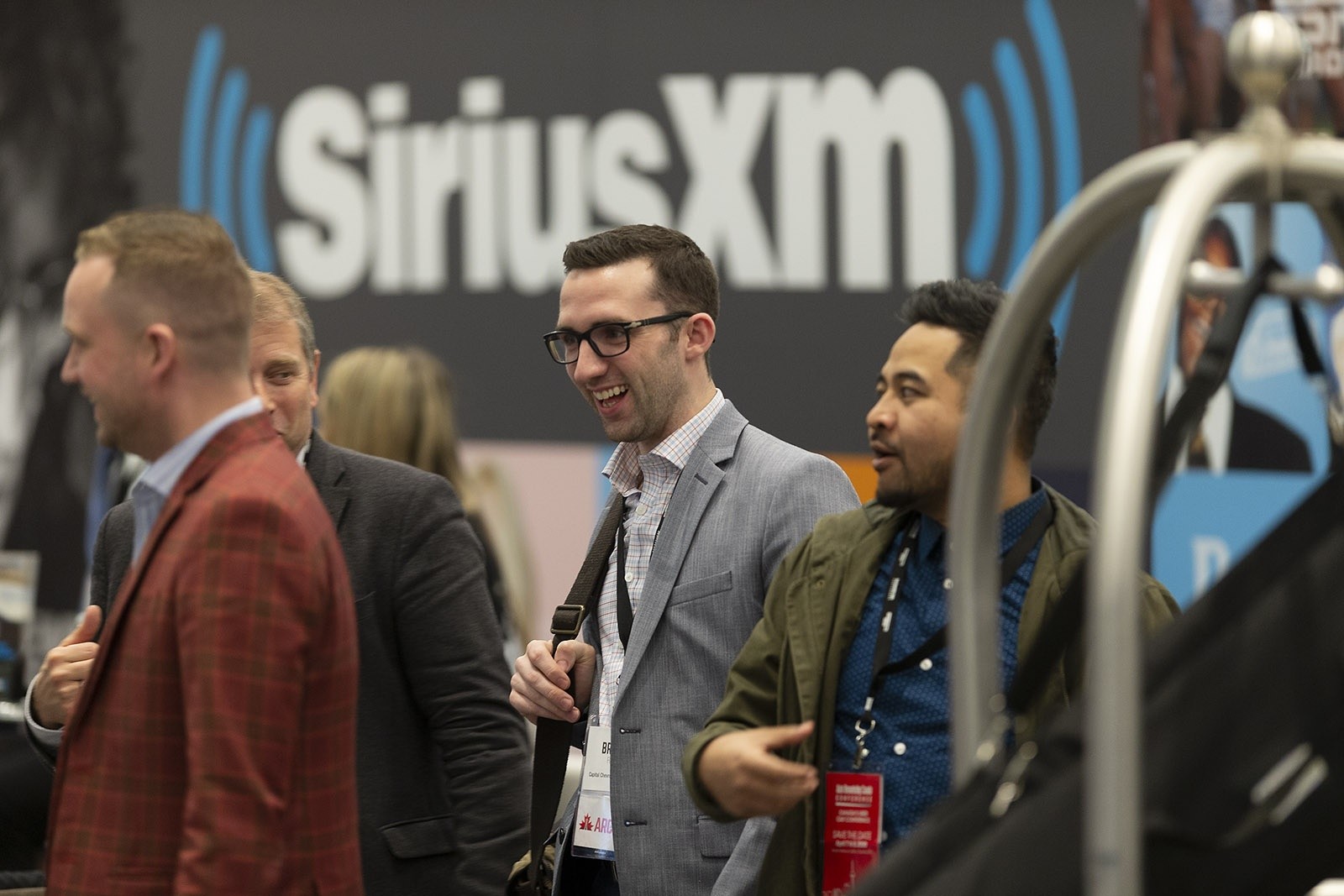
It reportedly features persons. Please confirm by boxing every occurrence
[1160,214,1315,472]
[673,278,1191,896]
[0,205,537,896]
[511,223,863,895]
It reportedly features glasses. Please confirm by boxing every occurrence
[542,312,694,364]
